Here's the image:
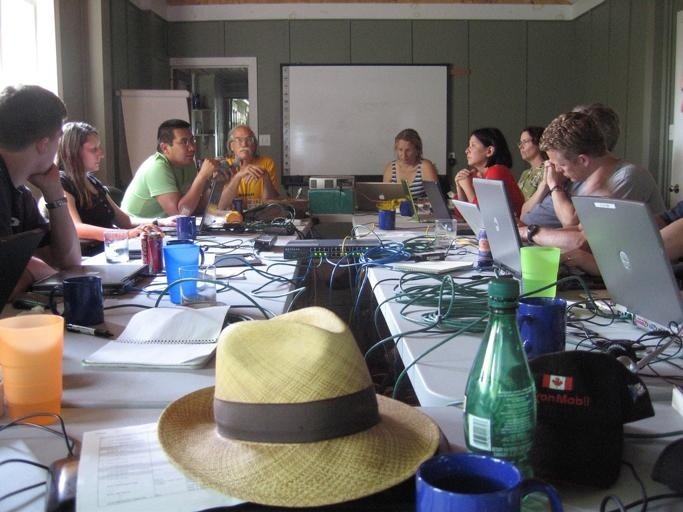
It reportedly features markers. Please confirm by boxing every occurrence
[13,298,50,310]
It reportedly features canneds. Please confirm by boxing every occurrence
[478,227,492,266]
[231,198,242,212]
[140,232,163,274]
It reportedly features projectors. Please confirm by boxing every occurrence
[308,175,355,190]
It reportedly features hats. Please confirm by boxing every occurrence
[156,307,441,507]
[529,350,655,493]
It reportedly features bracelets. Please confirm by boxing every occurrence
[152,218,157,226]
[45,197,67,209]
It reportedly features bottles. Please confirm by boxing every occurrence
[460,277,538,503]
[193,94,200,110]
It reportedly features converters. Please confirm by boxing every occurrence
[254,234,278,251]
[414,252,446,263]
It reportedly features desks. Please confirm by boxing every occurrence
[352,214,682,406]
[1,400,682,511]
[0,213,312,407]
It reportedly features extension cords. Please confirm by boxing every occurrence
[271,218,302,226]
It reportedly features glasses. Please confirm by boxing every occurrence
[176,136,198,146]
[232,136,254,143]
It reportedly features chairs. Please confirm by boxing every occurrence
[307,188,355,213]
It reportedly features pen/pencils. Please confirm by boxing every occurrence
[66,323,114,338]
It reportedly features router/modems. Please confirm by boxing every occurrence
[263,225,296,235]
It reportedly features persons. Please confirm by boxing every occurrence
[53,121,188,257]
[214,126,280,211]
[0,85,81,303]
[516,102,683,277]
[383,128,439,203]
[451,127,525,223]
[120,119,215,219]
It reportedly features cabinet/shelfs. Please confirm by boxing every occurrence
[189,92,219,160]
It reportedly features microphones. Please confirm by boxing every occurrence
[305,211,321,225]
[636,324,683,369]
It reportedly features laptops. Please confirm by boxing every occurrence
[160,176,218,236]
[113,235,171,259]
[355,182,405,212]
[0,226,47,317]
[422,181,475,235]
[451,199,484,241]
[401,178,435,223]
[472,177,586,281]
[31,265,150,295]
[570,196,683,329]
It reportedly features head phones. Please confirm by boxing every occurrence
[607,340,640,376]
[294,205,310,218]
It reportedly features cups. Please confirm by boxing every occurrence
[377,209,396,230]
[166,240,205,269]
[247,198,262,210]
[179,265,217,308]
[0,313,66,428]
[412,454,564,511]
[515,295,567,357]
[398,200,413,217]
[47,274,105,327]
[175,215,196,241]
[434,216,457,253]
[102,228,131,264]
[161,244,200,306]
[519,246,562,299]
